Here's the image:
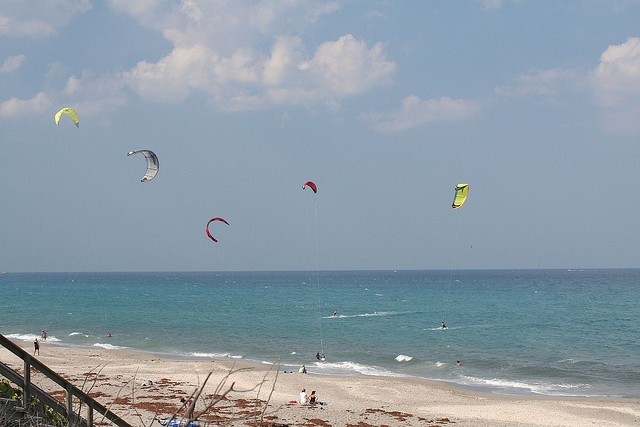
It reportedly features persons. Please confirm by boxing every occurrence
[315,352,320,360]
[180,397,195,419]
[441,321,446,328]
[300,388,307,404]
[308,390,317,405]
[456,360,460,365]
[40,329,46,339]
[299,363,306,372]
[33,338,40,355]
[321,353,326,360]
